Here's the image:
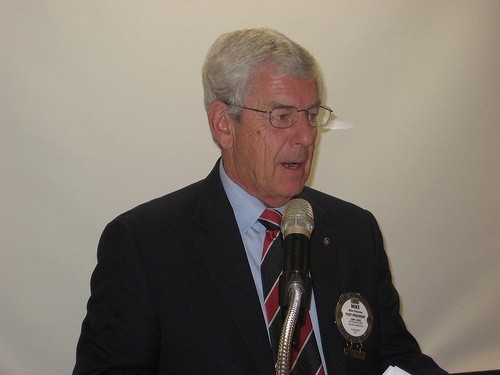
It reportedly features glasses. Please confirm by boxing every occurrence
[223,99,333,128]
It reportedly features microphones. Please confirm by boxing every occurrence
[278,199,314,350]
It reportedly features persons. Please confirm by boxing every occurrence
[72,28,449,375]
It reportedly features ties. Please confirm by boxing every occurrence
[258,208,326,375]
